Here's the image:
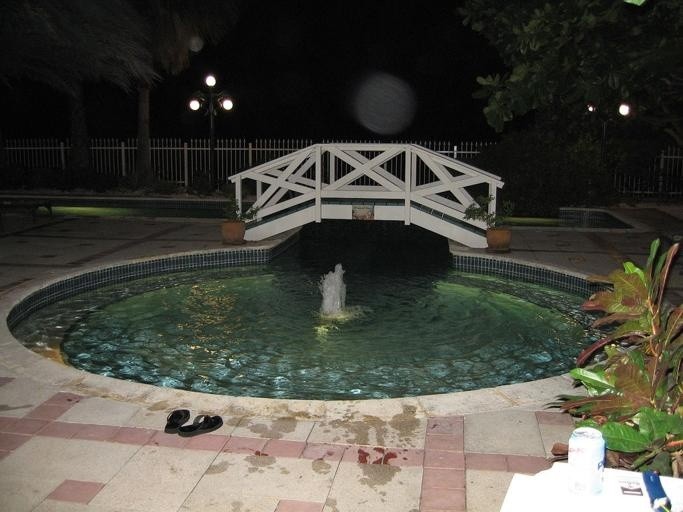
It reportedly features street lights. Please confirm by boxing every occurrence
[588,97,634,172]
[187,71,235,194]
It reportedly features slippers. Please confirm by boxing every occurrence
[178,414,223,437]
[164,409,190,433]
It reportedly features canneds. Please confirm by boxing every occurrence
[569,426,604,496]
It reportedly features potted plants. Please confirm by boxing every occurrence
[219,194,245,244]
[461,191,514,250]
